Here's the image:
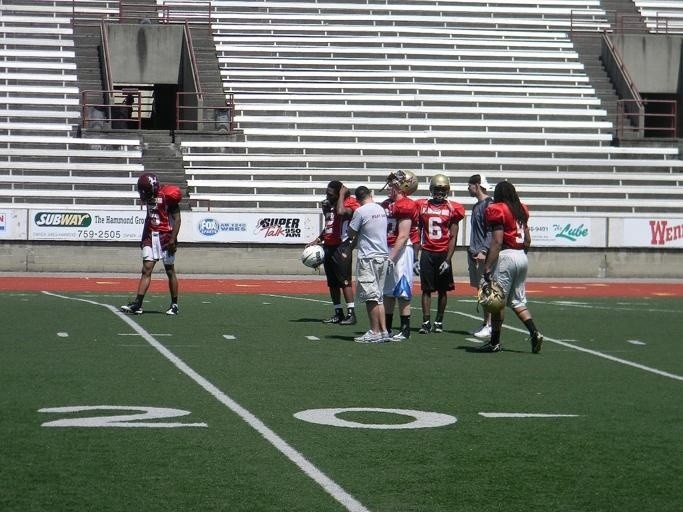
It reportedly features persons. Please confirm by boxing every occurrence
[412,174,465,334]
[121,174,182,314]
[305,181,361,325]
[380,170,420,342]
[468,174,495,337]
[477,181,543,353]
[381,186,395,209]
[347,186,390,343]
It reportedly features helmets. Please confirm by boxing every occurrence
[302,245,325,268]
[138,173,159,200]
[430,174,450,200]
[478,272,505,313]
[387,170,417,195]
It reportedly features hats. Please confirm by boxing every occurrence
[469,175,490,188]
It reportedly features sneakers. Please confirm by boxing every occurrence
[481,342,500,352]
[530,330,543,352]
[474,324,492,337]
[353,329,410,344]
[323,313,356,325]
[419,320,443,334]
[121,302,143,314]
[166,304,179,315]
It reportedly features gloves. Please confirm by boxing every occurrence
[413,259,420,275]
[439,261,450,275]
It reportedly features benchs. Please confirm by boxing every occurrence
[0,0,165,210]
